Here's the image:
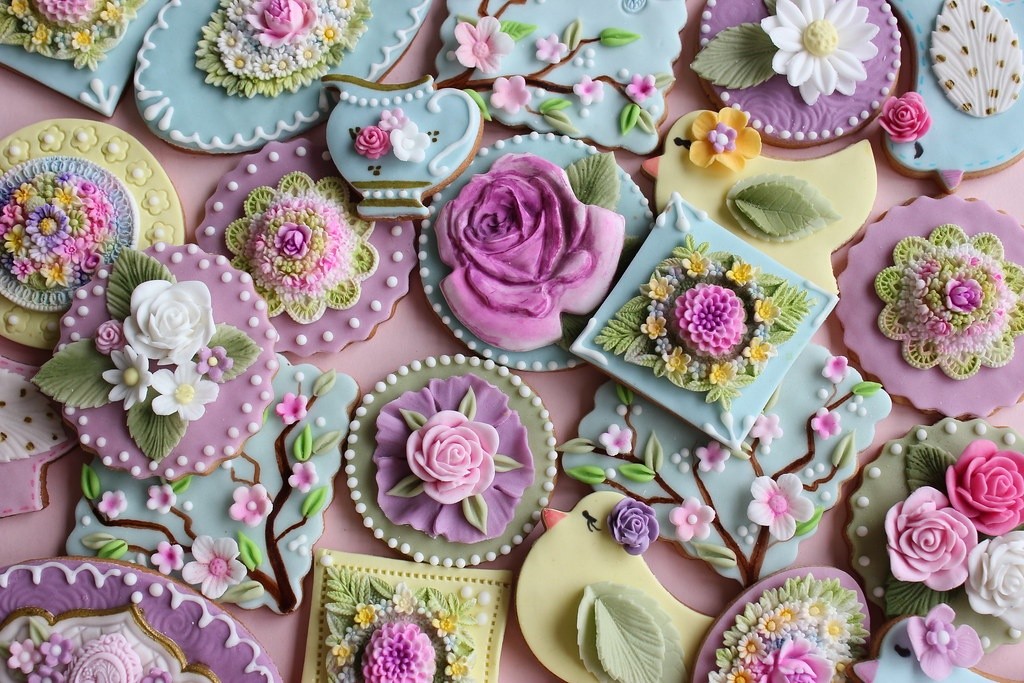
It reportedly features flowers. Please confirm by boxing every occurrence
[1,1,1024,683]
